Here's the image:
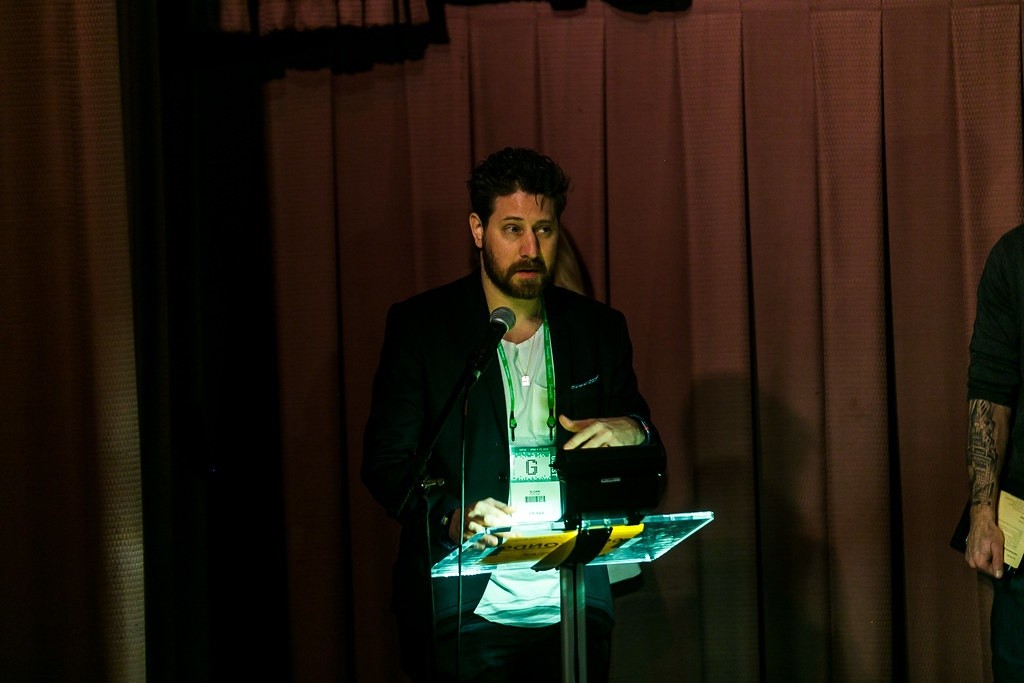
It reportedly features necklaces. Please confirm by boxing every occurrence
[505,298,540,386]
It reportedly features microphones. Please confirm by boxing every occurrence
[468,306,516,390]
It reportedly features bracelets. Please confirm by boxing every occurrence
[630,414,650,445]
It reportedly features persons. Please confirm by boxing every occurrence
[364,148,667,683]
[964,222,1024,683]
